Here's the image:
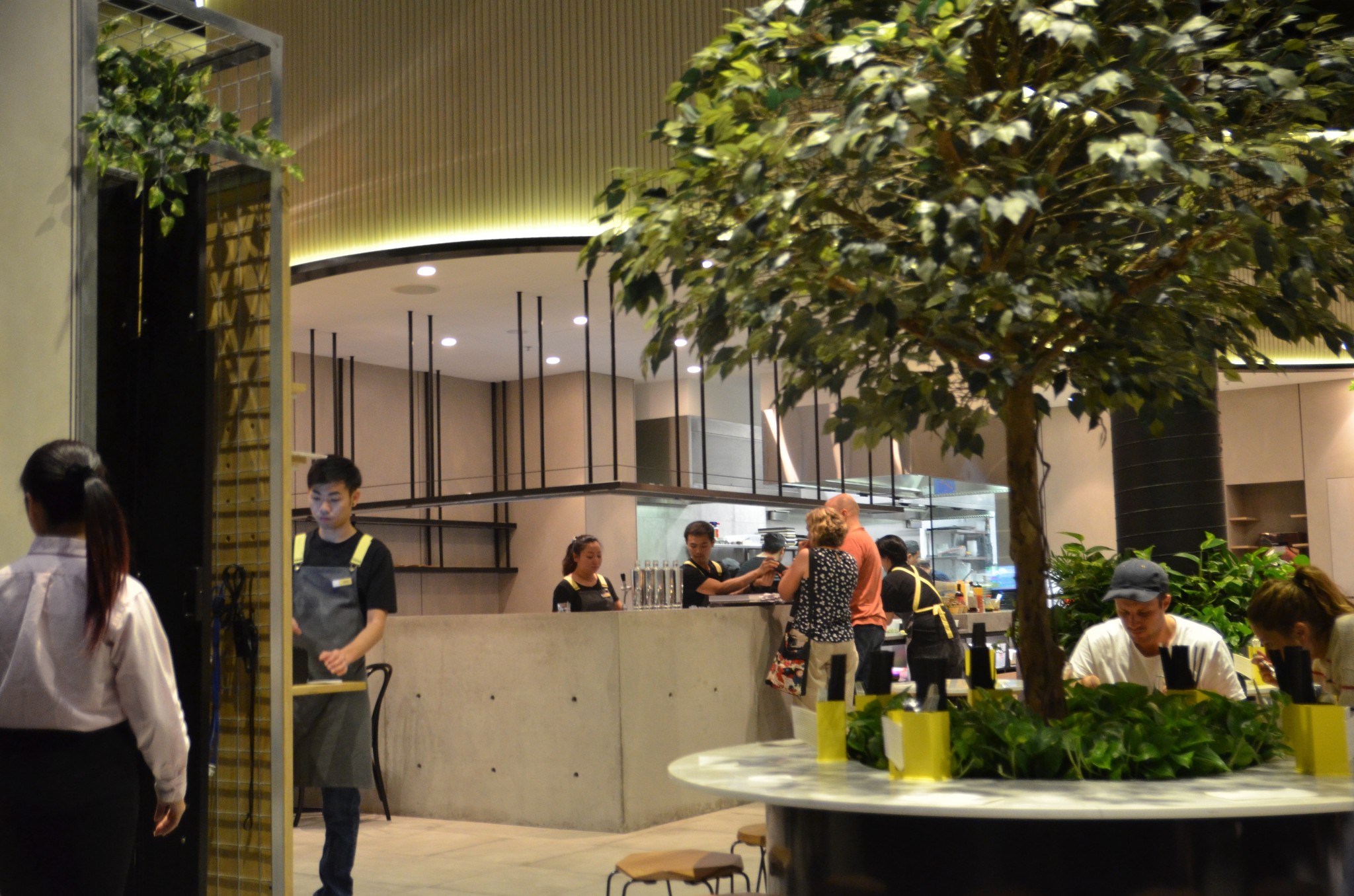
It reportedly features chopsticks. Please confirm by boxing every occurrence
[1159,644,1205,690]
[1268,645,1315,705]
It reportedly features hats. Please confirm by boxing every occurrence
[764,533,789,547]
[1101,559,1168,602]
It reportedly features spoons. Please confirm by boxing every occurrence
[903,698,922,712]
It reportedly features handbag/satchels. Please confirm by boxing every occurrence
[765,632,811,697]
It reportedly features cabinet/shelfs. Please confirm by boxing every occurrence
[1290,514,1309,547]
[1230,516,1258,549]
[926,529,990,560]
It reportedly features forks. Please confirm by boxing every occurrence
[817,689,828,702]
[924,695,940,711]
[854,682,865,695]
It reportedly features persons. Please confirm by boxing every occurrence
[905,539,921,683]
[0,439,189,896]
[1277,541,1296,561]
[1246,565,1354,708]
[825,494,887,696]
[681,520,779,609]
[918,560,951,582]
[552,534,623,613]
[735,531,787,593]
[1063,559,1246,702]
[777,507,859,713]
[288,457,397,896]
[875,535,966,713]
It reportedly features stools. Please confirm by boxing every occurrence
[730,823,768,894]
[605,850,750,895]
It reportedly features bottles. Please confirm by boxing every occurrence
[632,560,684,611]
[955,580,983,613]
[984,595,994,612]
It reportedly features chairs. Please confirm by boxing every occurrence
[293,663,392,827]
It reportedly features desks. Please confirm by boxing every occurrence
[667,742,1354,896]
[293,680,367,696]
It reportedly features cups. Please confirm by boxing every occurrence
[941,596,968,614]
[557,603,571,613]
[967,540,977,556]
[719,535,761,546]
[991,599,1000,612]
